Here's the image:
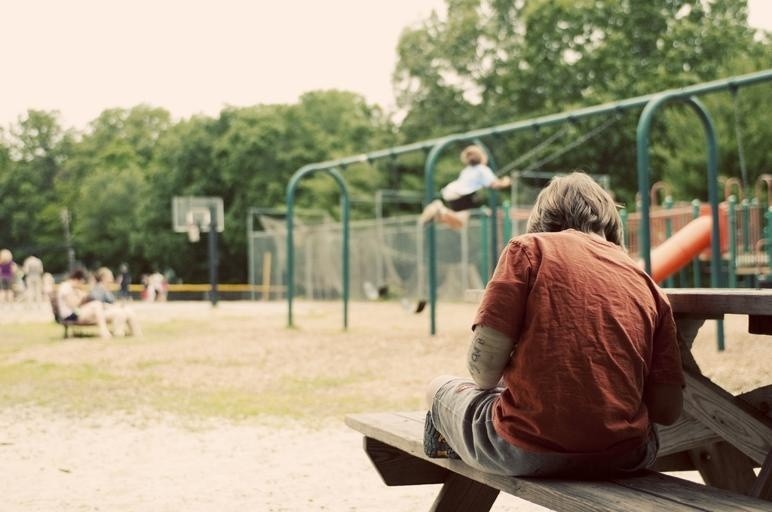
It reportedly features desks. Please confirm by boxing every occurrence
[654,287,770,499]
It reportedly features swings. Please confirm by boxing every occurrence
[729,71,772,288]
[440,112,624,212]
[390,147,431,313]
[339,152,388,301]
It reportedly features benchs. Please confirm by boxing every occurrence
[46,289,111,340]
[344,409,770,511]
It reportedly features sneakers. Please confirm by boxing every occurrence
[417,200,442,227]
[424,409,461,460]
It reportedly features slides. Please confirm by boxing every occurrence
[634,215,713,284]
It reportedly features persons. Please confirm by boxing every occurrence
[0,247,170,339]
[423,167,688,480]
[419,143,511,232]
